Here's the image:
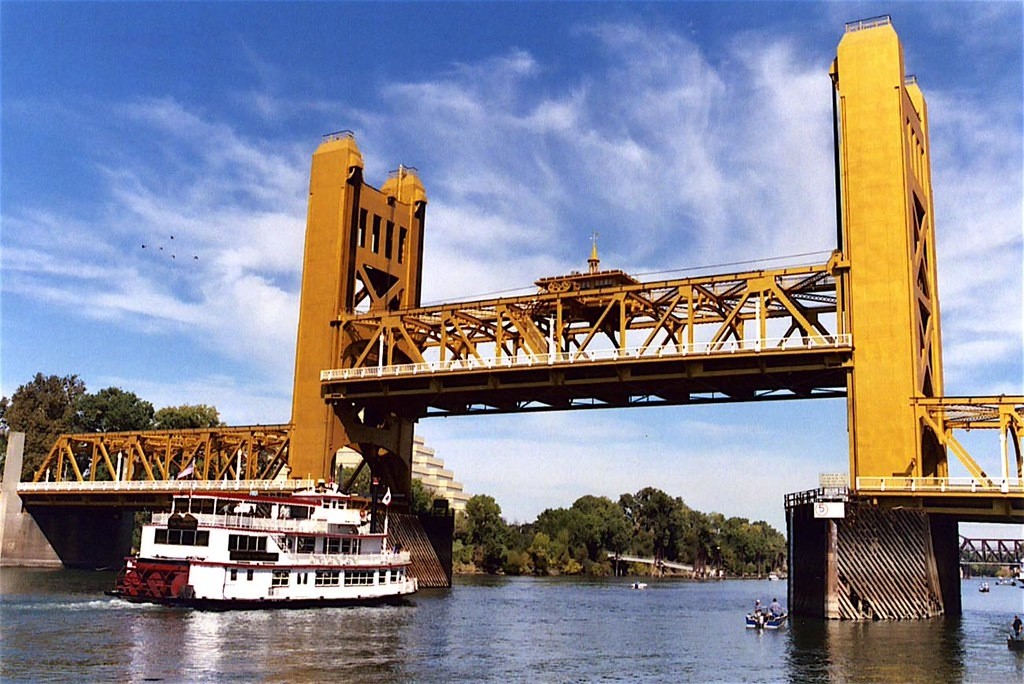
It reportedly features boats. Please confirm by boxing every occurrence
[745,605,788,630]
[768,574,780,581]
[103,470,422,613]
[630,580,648,590]
[979,582,989,592]
[1006,638,1024,651]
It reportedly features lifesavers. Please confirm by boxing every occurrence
[360,506,364,517]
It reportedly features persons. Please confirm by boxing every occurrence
[1010,616,1022,640]
[218,502,291,519]
[754,598,781,636]
[393,541,402,554]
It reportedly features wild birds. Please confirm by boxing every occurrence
[141,236,199,260]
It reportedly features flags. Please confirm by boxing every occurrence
[382,486,392,507]
[178,463,193,478]
